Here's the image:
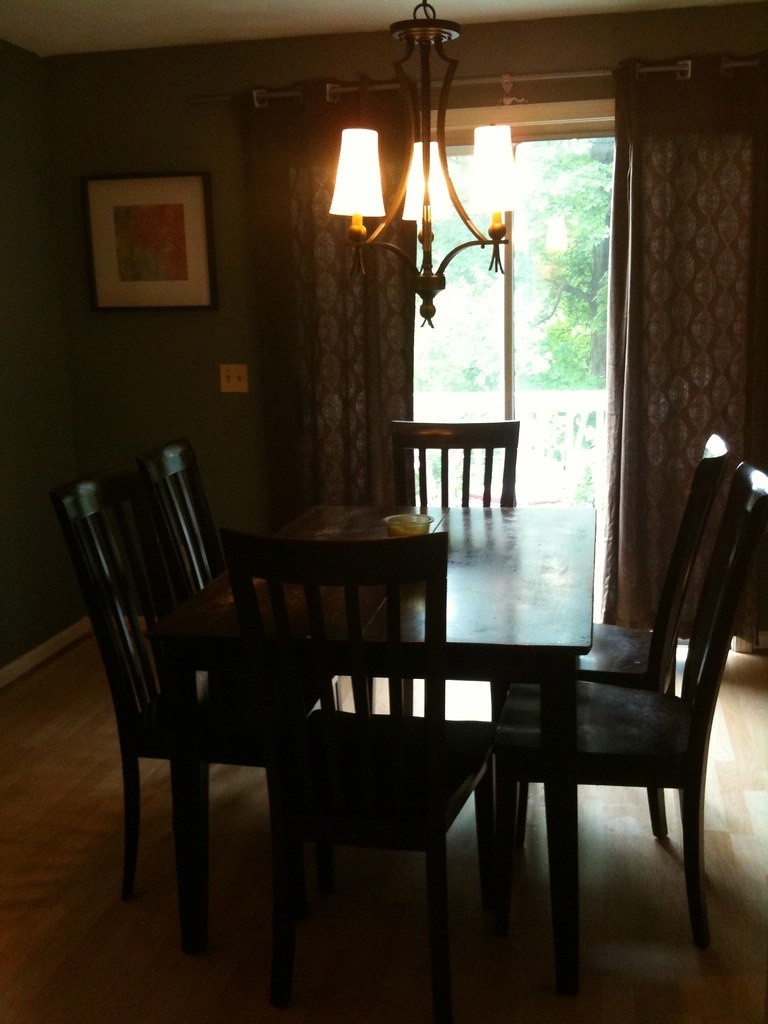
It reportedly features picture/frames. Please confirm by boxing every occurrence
[83,168,218,312]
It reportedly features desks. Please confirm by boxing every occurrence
[142,505,613,996]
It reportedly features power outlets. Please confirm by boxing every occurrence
[221,364,248,393]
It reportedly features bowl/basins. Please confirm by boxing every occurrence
[382,513,434,537]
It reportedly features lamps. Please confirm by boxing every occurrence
[329,0,530,330]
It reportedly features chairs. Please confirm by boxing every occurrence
[60,419,768,1024]
